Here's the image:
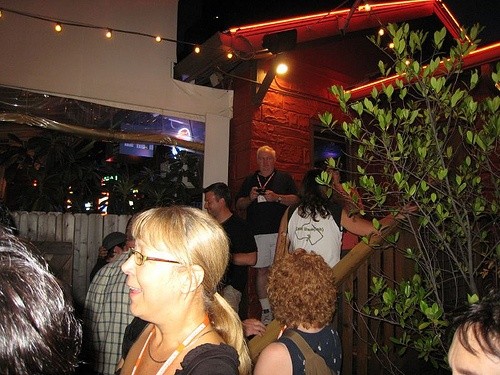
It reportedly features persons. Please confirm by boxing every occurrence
[447,298,500,375]
[204,182,258,315]
[314,153,363,258]
[0,224,83,375]
[83,212,267,375]
[114,206,252,375]
[236,145,302,327]
[273,170,417,265]
[253,248,343,375]
[89,232,125,284]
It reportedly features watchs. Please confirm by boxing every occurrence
[278,195,282,203]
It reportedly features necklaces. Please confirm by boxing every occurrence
[147,326,216,363]
[129,315,210,375]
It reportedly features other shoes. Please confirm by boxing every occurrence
[261,309,273,326]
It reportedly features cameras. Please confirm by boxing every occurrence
[256,188,266,195]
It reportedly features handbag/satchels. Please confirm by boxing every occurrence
[283,330,333,374]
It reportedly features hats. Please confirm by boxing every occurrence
[102,231,126,250]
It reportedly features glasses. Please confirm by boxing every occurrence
[129,247,179,266]
